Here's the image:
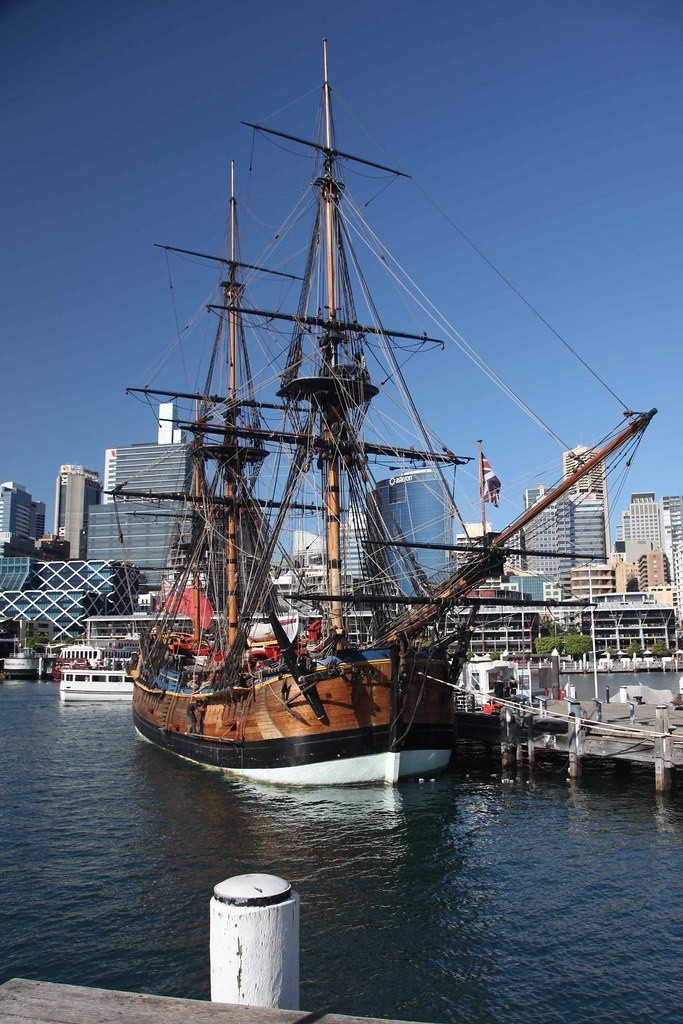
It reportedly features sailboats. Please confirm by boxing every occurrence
[103,34,658,788]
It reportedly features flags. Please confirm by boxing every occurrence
[480,453,501,508]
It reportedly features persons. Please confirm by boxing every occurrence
[494,676,504,699]
[510,676,517,695]
[84,676,89,681]
[68,659,103,669]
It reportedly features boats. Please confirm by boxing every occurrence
[58,668,134,700]
